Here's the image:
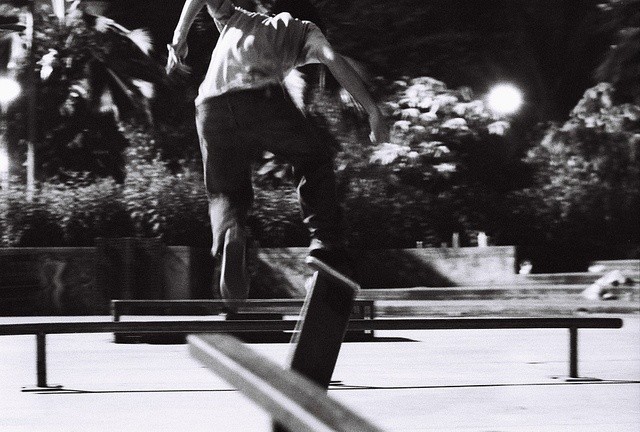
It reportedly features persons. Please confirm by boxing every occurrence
[166,0,384,309]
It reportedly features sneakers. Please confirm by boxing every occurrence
[308,237,362,293]
[219,225,251,312]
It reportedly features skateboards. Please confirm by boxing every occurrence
[273,272,355,432]
